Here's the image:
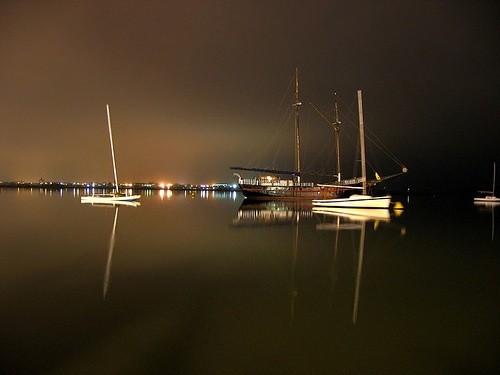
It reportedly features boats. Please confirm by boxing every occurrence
[475,163,500,201]
[86,200,141,296]
[79,104,142,202]
[310,86,392,208]
[312,205,390,320]
[474,201,500,239]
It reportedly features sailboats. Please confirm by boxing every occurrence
[233,197,415,326]
[235,65,412,200]
